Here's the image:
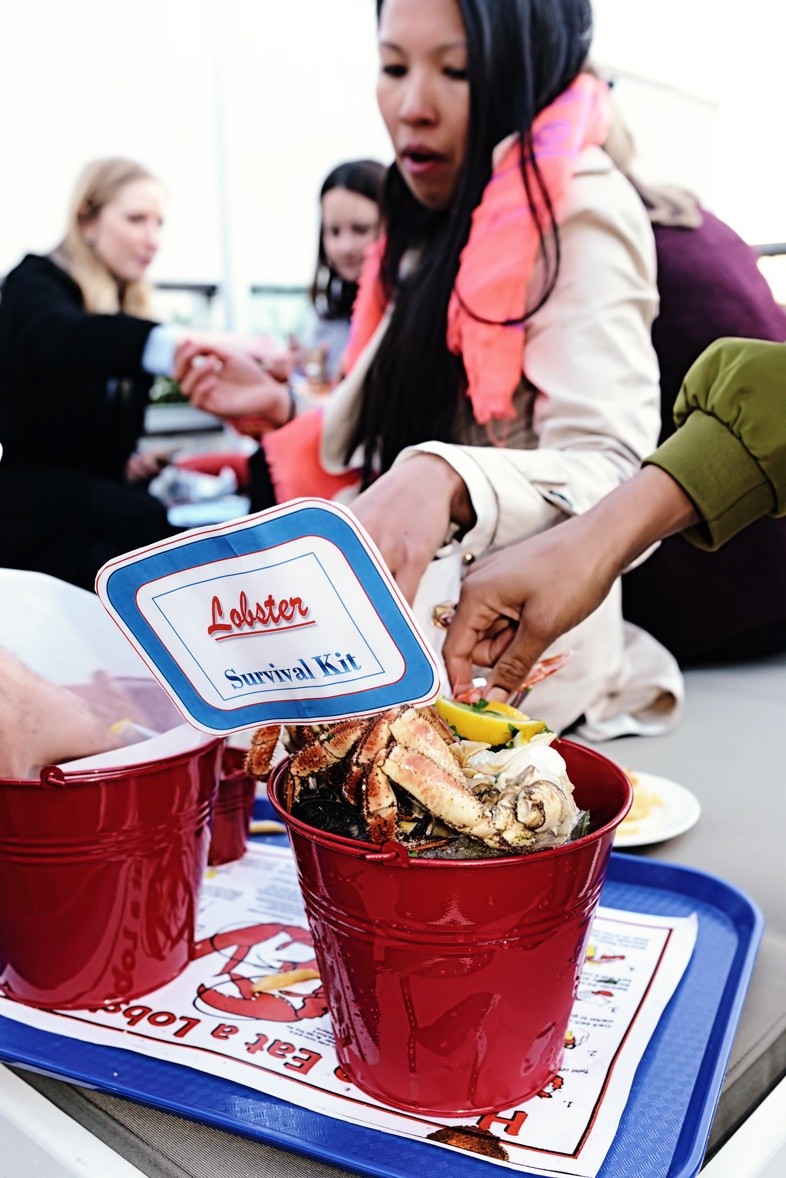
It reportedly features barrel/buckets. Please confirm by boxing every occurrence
[266,735,634,1119]
[0,736,257,1010]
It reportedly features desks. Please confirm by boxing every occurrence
[0,663,784,1178]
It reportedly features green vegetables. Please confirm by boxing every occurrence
[449,698,553,748]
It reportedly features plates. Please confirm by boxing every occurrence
[606,769,700,850]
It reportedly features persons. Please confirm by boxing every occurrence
[286,156,386,391]
[611,115,786,674]
[444,336,786,712]
[172,0,664,735]
[0,160,292,593]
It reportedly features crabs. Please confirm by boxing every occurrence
[244,704,580,851]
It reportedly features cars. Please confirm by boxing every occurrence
[136,327,325,445]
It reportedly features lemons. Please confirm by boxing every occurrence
[436,696,547,747]
[482,701,529,721]
